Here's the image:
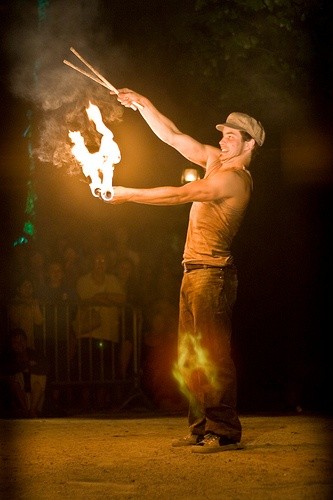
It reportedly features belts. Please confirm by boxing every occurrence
[183,262,220,270]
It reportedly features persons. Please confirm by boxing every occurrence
[99,88,266,453]
[0,207,183,418]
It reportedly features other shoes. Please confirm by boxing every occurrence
[171,433,203,446]
[191,434,244,453]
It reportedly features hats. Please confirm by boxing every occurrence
[216,112,266,147]
